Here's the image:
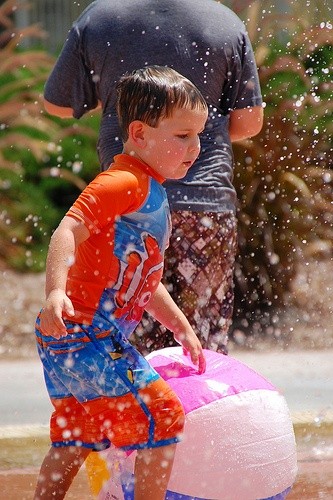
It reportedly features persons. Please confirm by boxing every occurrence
[43,0,265,356]
[35,65,210,500]
